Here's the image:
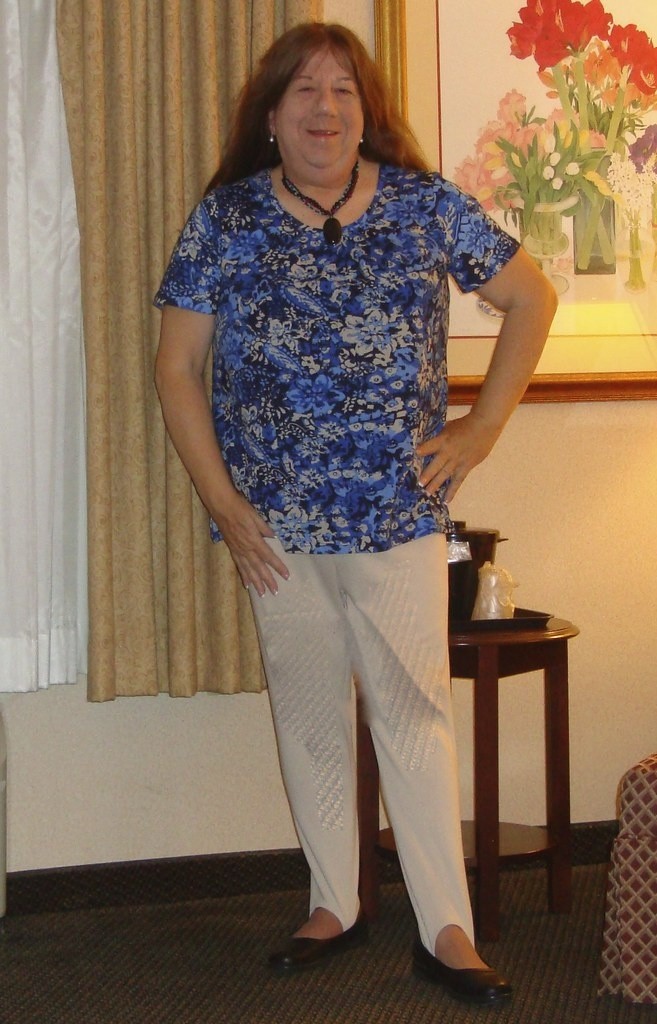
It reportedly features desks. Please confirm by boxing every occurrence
[348,621,579,936]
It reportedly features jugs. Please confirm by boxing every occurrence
[443,520,501,624]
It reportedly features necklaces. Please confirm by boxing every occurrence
[281,161,359,245]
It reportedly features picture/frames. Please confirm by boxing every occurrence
[371,1,656,407]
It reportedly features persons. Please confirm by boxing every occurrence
[153,23,558,1000]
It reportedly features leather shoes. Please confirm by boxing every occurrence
[267,919,368,970]
[412,937,514,1004]
